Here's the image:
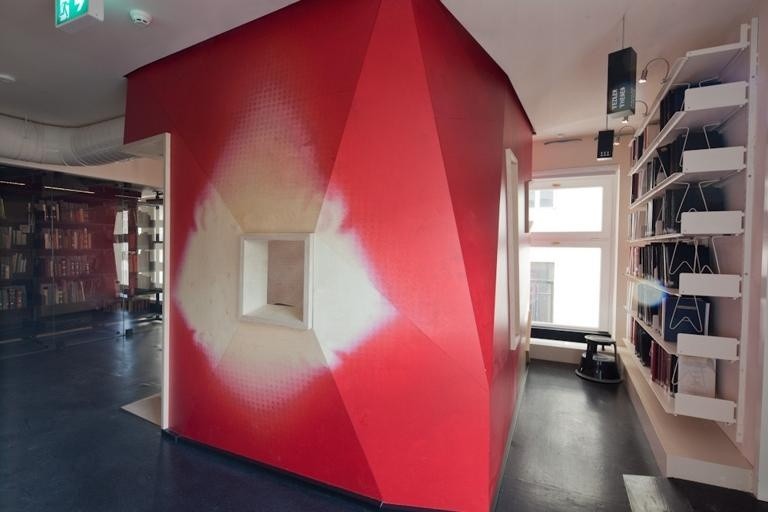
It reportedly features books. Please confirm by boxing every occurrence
[628,90,725,395]
[0,200,93,310]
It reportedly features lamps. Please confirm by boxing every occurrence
[606,13,638,119]
[638,57,669,85]
[621,100,651,124]
[613,125,635,145]
[597,116,615,161]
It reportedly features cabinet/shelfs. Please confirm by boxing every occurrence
[615,18,768,504]
[0,163,163,328]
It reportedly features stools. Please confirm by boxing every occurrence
[574,334,625,385]
